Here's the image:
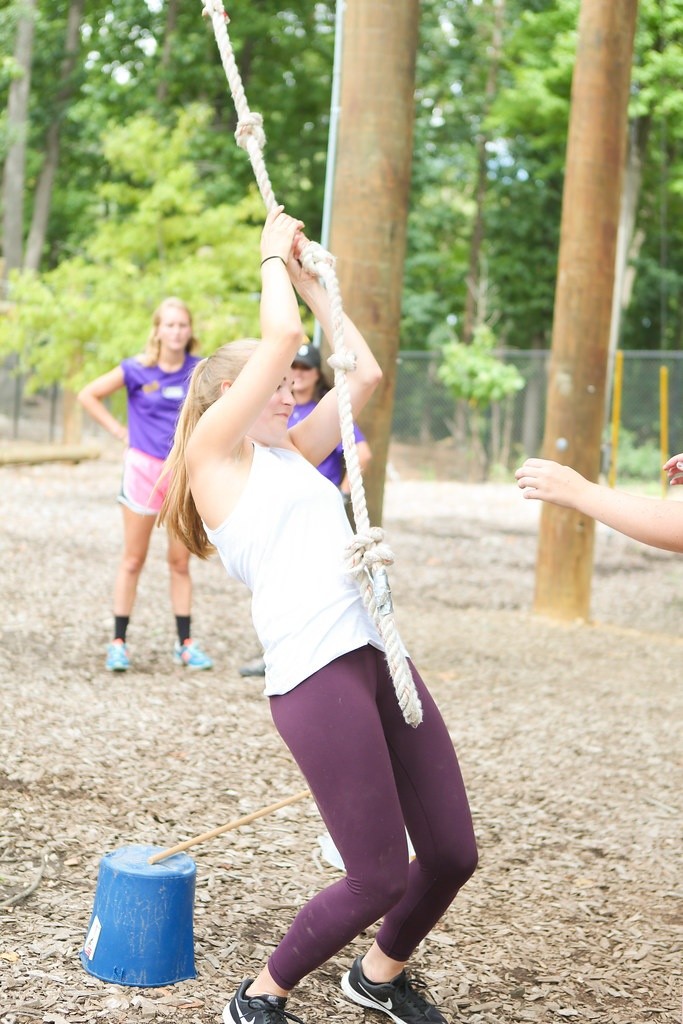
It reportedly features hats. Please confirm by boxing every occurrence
[294,344,320,368]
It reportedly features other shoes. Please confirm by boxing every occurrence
[239,661,266,676]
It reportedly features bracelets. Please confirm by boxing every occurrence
[261,255,288,266]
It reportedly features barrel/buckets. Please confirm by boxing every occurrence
[80,841,198,987]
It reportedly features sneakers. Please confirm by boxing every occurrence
[222,979,304,1024]
[341,952,448,1024]
[173,638,213,670]
[104,638,129,671]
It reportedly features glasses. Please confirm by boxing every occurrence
[291,362,310,370]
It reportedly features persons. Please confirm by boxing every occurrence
[157,206,479,1024]
[76,297,213,672]
[515,454,683,553]
[242,342,372,675]
